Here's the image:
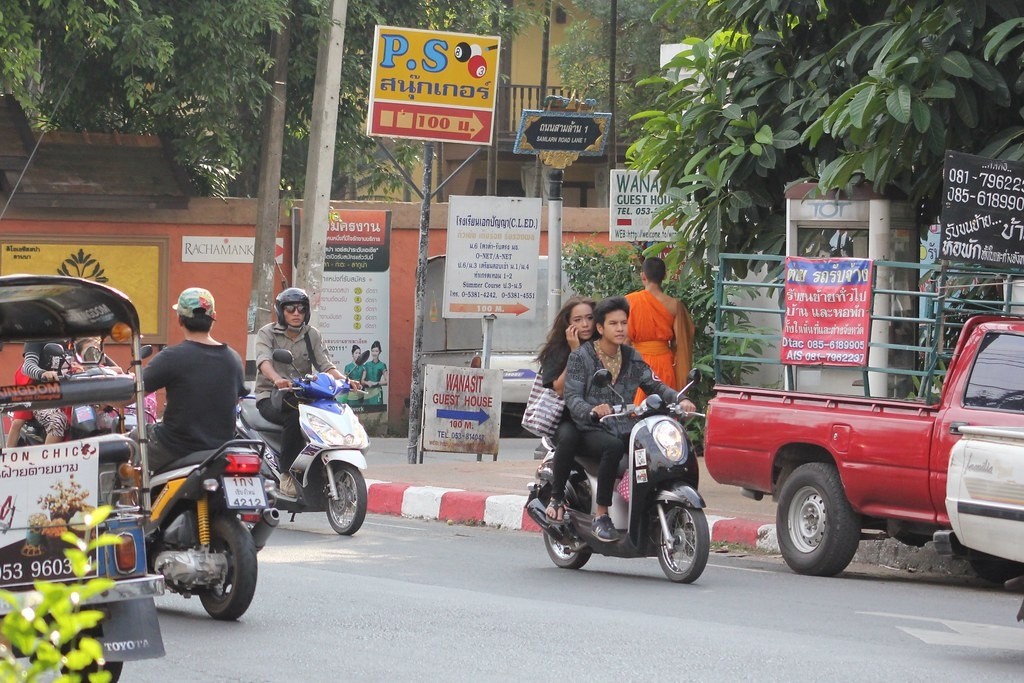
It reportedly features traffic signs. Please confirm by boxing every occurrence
[365,25,501,152]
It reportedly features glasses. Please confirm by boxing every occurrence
[282,303,307,314]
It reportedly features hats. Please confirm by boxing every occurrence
[171,287,217,322]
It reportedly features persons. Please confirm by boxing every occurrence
[625,257,694,418]
[345,342,388,405]
[563,296,697,542]
[7,337,124,448]
[536,297,598,525]
[254,287,362,498]
[131,287,252,470]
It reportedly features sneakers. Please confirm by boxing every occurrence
[279,476,298,498]
[591,513,621,543]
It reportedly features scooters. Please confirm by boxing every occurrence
[9,342,153,447]
[240,348,378,535]
[106,403,280,621]
[519,365,717,585]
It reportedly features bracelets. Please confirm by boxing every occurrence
[377,382,379,386]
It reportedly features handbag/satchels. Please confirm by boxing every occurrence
[520,366,566,440]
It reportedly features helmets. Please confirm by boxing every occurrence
[274,287,311,327]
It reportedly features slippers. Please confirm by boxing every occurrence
[546,500,567,525]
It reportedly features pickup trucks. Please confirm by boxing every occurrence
[701,316,1024,580]
